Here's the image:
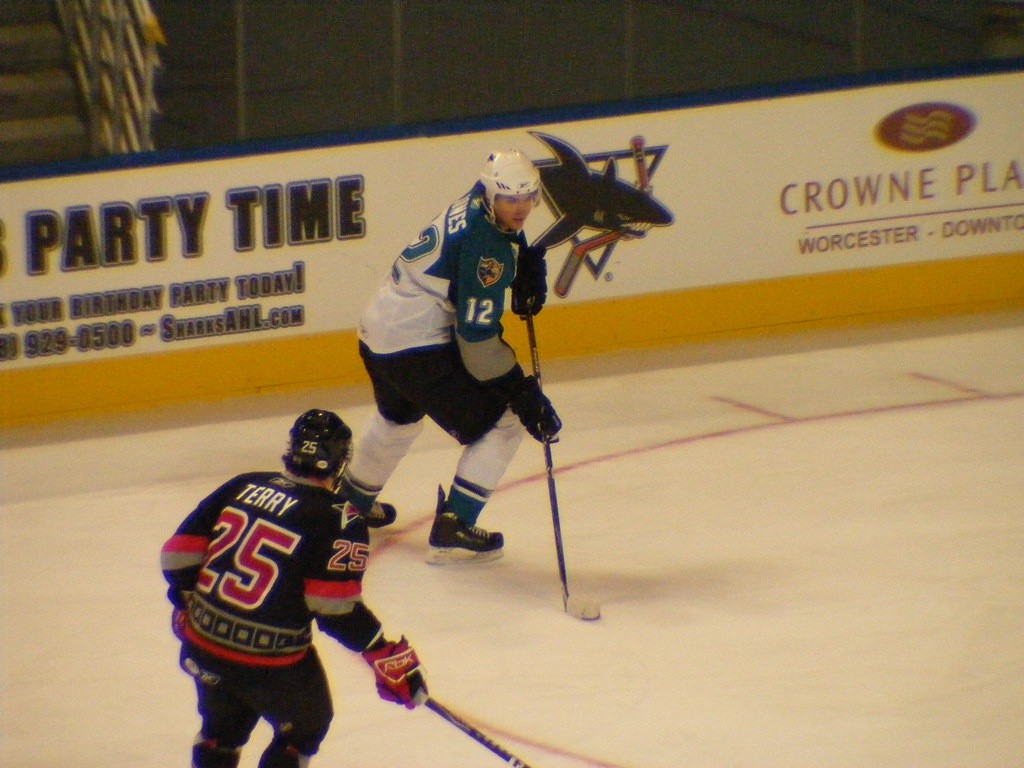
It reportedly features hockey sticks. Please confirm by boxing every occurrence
[525,308,603,622]
[421,692,531,768]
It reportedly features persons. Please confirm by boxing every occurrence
[157,407,432,768]
[335,149,563,569]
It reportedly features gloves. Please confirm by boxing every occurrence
[363,634,429,711]
[508,247,547,320]
[171,605,189,641]
[502,374,563,444]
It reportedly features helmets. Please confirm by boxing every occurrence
[282,409,352,481]
[481,150,540,203]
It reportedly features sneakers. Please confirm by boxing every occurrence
[425,485,505,565]
[337,476,397,528]
